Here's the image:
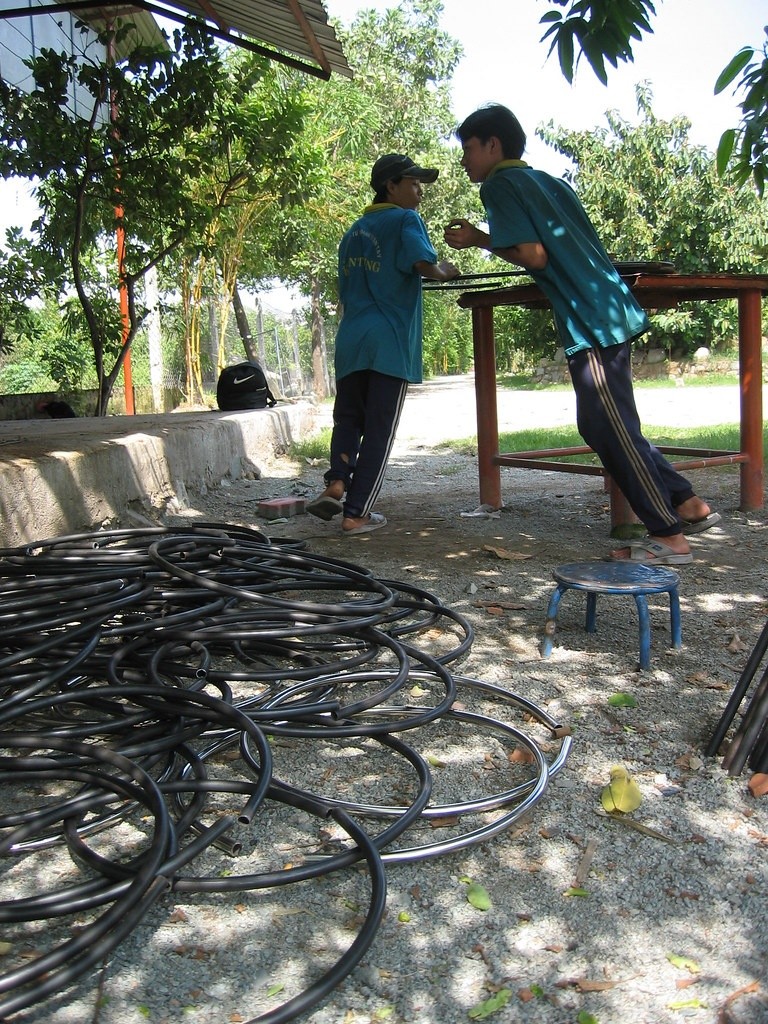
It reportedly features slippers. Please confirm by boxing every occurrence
[604,536,694,565]
[305,496,343,521]
[678,512,721,535]
[343,512,387,535]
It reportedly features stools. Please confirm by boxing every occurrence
[541,561,681,671]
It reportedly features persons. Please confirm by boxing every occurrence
[306,154,461,535]
[443,106,720,565]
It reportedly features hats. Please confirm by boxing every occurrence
[369,153,439,191]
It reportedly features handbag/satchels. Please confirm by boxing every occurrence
[217,361,276,410]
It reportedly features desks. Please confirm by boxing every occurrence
[456,272,768,540]
